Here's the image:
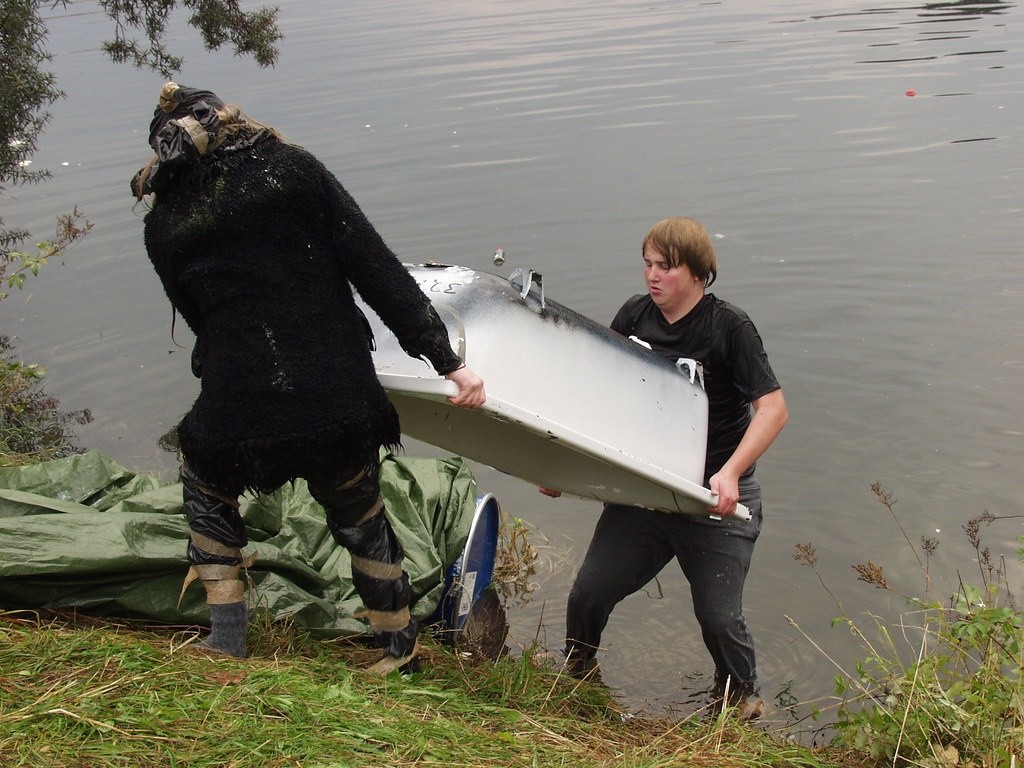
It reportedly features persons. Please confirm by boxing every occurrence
[539,217,788,696]
[131,82,488,679]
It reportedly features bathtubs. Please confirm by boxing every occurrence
[348,263,752,542]
